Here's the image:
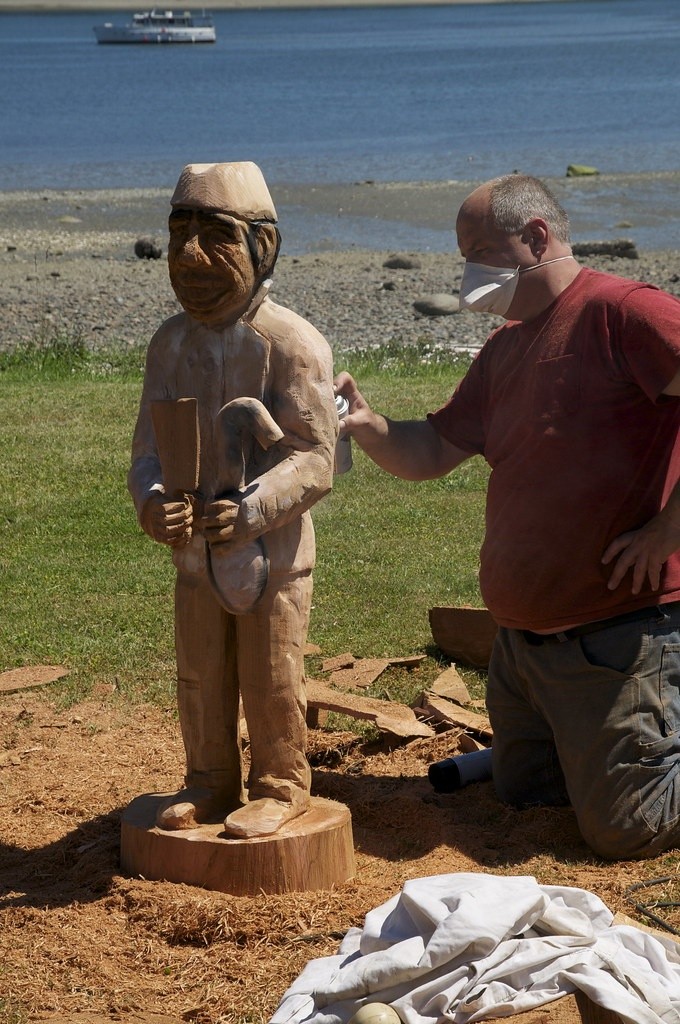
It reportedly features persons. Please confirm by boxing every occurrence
[333,176,680,854]
[128,164,339,838]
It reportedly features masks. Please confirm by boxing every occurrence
[457,256,576,316]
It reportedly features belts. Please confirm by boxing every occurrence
[517,601,680,647]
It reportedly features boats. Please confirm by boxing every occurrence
[92,8,217,44]
